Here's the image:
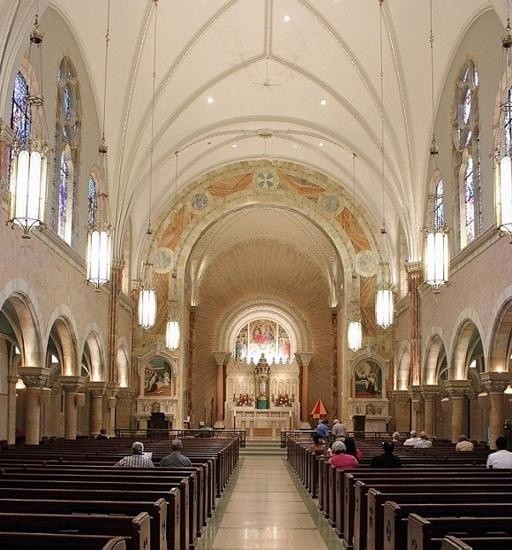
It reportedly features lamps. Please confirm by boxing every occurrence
[420,0,452,294]
[489,0,512,244]
[347,153,363,352]
[374,0,394,330]
[137,0,158,331]
[165,151,181,352]
[5,0,51,239]
[86,0,114,293]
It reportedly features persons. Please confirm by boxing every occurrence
[402,429,420,447]
[331,418,347,442]
[390,432,401,448]
[311,435,326,455]
[145,371,170,392]
[485,436,511,469]
[344,437,362,461]
[355,368,379,394]
[329,443,359,470]
[95,429,109,440]
[160,439,193,467]
[113,441,155,468]
[456,435,473,451]
[315,419,330,441]
[413,431,432,449]
[369,441,401,469]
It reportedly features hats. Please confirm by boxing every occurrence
[384,441,395,451]
[331,441,346,453]
[132,441,143,453]
[168,439,183,449]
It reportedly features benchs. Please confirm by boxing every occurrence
[0,429,246,550]
[281,429,512,550]
